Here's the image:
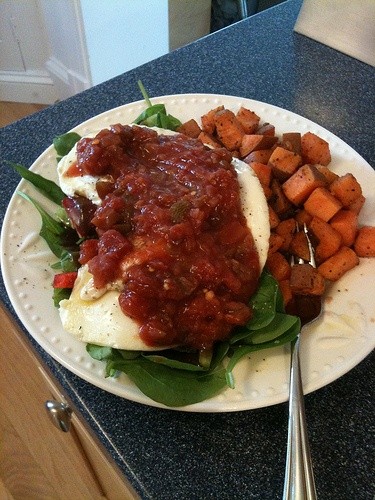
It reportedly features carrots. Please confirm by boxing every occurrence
[178,104,374,308]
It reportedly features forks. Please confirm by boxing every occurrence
[282,219,324,500]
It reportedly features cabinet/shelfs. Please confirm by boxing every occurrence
[0,0,375,500]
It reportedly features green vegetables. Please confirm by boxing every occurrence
[3,78,302,409]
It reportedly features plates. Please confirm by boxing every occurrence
[1,93,375,413]
